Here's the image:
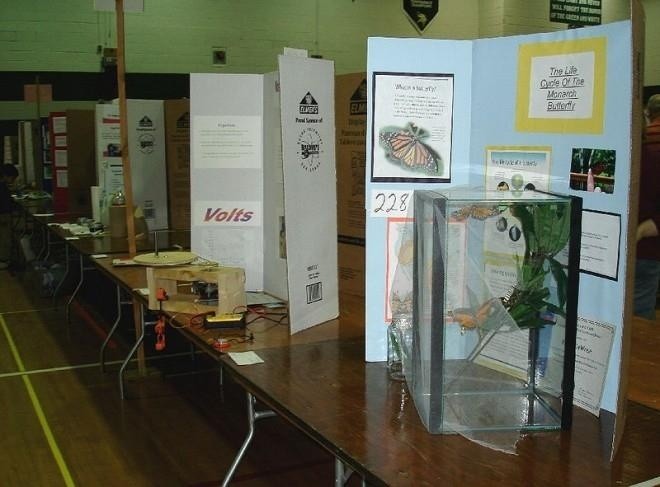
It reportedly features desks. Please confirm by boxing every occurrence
[0,178,659,486]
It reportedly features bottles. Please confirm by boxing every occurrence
[385,382,410,434]
[75,216,109,235]
[100,190,108,217]
[142,200,157,230]
[383,296,414,379]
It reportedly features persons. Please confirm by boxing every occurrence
[632,94,659,323]
[0,162,25,270]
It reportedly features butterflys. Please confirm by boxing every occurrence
[452,296,498,335]
[450,205,496,222]
[380,121,441,177]
